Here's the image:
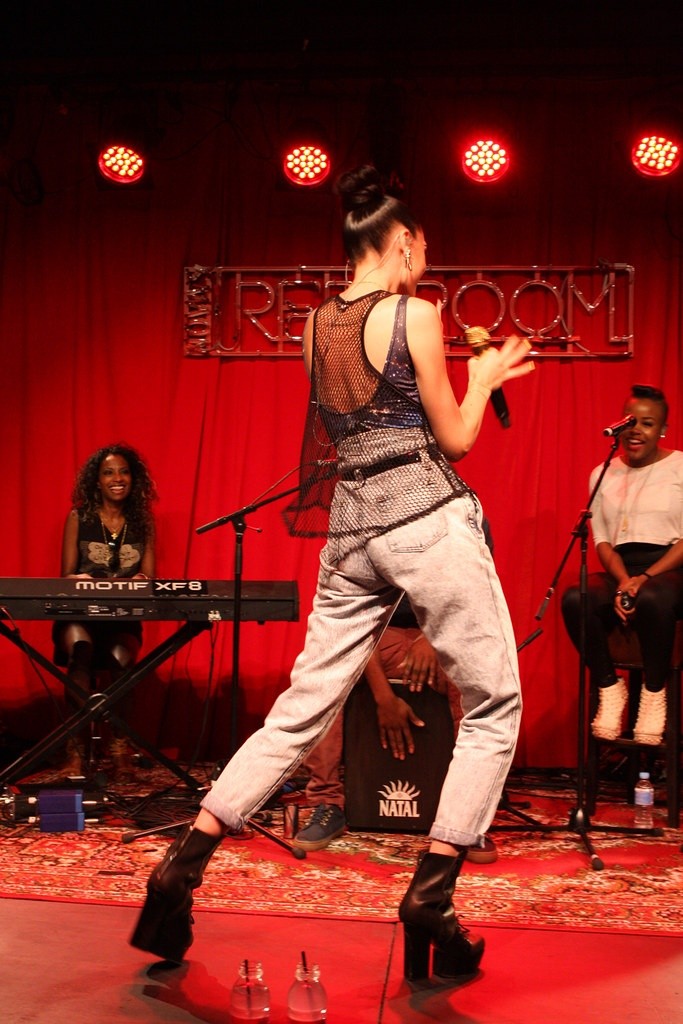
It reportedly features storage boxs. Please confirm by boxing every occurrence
[37,790,86,832]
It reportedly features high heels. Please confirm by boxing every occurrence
[397,849,485,985]
[132,821,227,962]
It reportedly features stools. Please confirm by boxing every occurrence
[575,614,683,826]
[53,650,152,771]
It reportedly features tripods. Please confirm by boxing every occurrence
[487,435,666,872]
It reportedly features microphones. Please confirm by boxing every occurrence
[311,458,341,467]
[465,326,511,428]
[603,415,637,435]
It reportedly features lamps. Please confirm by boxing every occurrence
[448,114,525,187]
[626,82,683,177]
[90,112,167,190]
[273,116,339,195]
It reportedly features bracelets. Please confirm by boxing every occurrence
[641,572,651,578]
[136,573,147,580]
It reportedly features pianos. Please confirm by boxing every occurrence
[0,578,302,796]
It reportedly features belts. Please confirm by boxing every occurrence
[341,447,438,481]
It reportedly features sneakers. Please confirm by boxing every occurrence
[294,802,346,852]
[465,836,498,863]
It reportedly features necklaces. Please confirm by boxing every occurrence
[99,511,127,554]
[622,446,657,539]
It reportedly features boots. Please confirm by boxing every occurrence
[108,655,137,782]
[70,642,94,775]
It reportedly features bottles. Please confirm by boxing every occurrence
[288,962,327,1024]
[634,772,653,836]
[230,961,269,1024]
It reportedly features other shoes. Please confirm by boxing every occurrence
[591,679,628,741]
[633,684,667,746]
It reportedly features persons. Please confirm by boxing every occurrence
[561,384,683,744]
[54,444,154,773]
[129,164,535,990]
[292,514,498,864]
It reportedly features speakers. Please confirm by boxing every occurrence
[341,676,456,833]
